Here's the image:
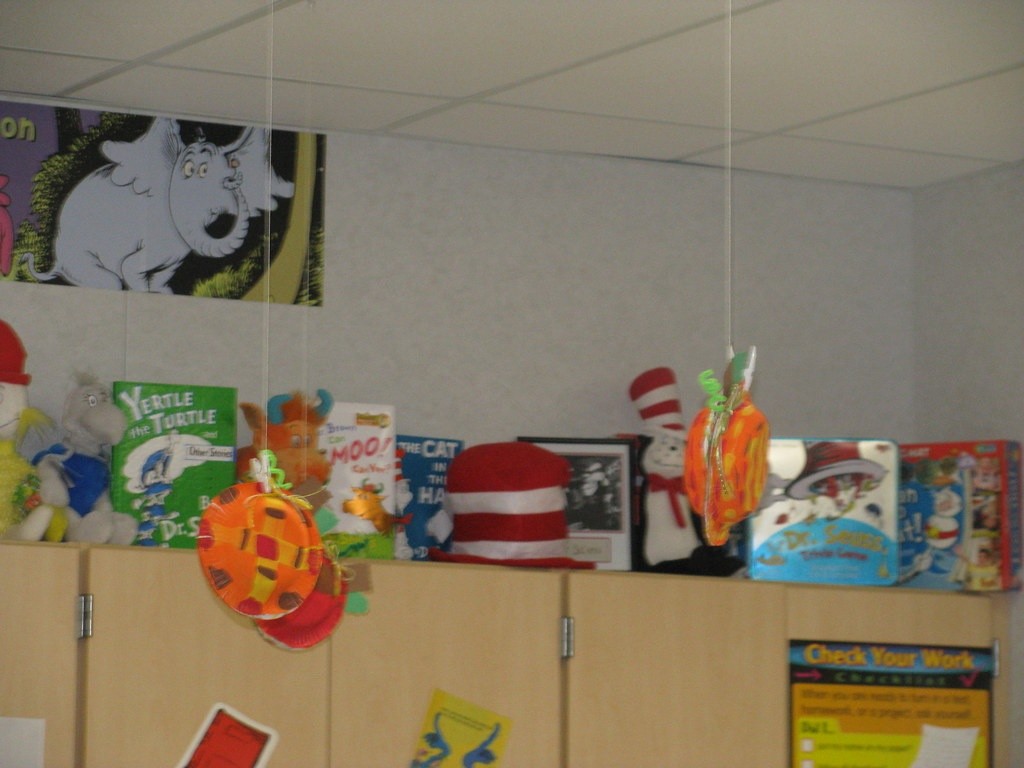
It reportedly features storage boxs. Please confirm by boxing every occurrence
[392,434,466,561]
[111,379,238,547]
[309,400,398,514]
[745,436,899,587]
[907,439,1024,591]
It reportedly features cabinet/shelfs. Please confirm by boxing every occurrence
[0,540,1012,768]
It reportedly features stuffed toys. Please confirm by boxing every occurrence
[0,315,53,544]
[29,383,138,544]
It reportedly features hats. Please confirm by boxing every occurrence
[254,554,348,651]
[425,441,597,568]
[198,482,324,620]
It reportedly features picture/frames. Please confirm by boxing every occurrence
[516,435,640,573]
